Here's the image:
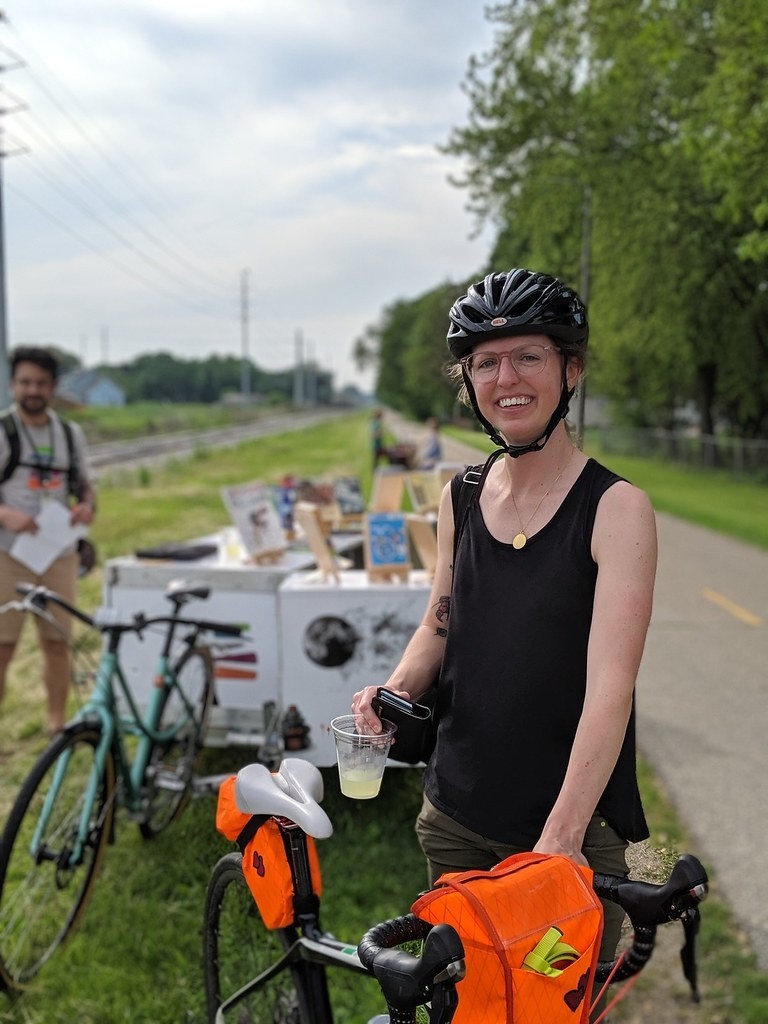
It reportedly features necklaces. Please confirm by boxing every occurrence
[506,448,575,550]
[21,411,55,490]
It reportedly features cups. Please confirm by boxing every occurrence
[330,713,398,800]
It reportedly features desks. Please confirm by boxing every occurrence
[273,570,434,768]
[106,525,365,745]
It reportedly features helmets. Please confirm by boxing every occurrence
[443,267,590,353]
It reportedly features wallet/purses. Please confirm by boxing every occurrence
[353,688,431,765]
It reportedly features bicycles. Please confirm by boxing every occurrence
[194,757,708,1024]
[0,579,252,996]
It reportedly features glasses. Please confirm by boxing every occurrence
[459,344,561,385]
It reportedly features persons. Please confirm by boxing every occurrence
[415,417,442,470]
[369,410,390,467]
[352,268,657,1024]
[0,347,97,739]
